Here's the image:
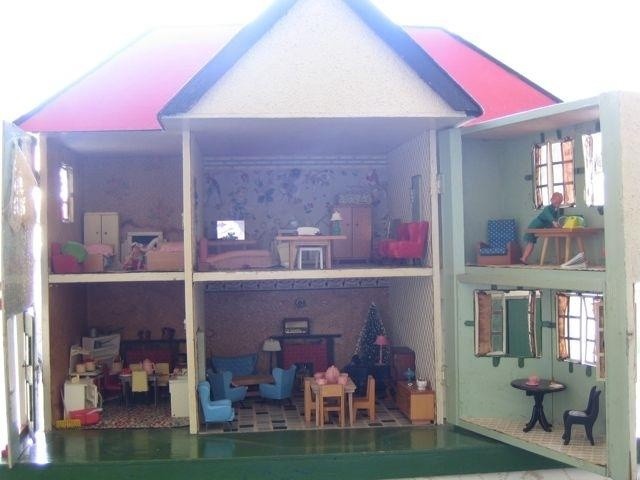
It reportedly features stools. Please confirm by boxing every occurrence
[298,247,324,270]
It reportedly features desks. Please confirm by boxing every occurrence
[276,235,348,269]
[209,239,256,253]
[71,368,107,394]
[523,227,600,266]
[117,376,159,407]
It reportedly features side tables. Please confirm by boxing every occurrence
[510,378,566,432]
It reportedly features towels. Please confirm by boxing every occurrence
[131,370,148,393]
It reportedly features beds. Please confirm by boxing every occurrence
[145,240,184,271]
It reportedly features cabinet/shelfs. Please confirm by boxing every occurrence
[83,211,120,267]
[396,380,435,425]
[125,340,187,373]
[169,376,190,418]
[81,333,121,368]
[391,346,415,381]
[267,334,342,378]
[330,203,373,265]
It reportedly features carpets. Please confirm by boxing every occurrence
[468,263,605,272]
[200,389,413,432]
[463,416,606,466]
[82,398,190,428]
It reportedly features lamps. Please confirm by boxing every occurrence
[261,338,282,374]
[373,334,390,365]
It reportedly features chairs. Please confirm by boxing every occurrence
[205,368,248,407]
[475,218,521,265]
[50,242,80,273]
[303,374,378,428]
[259,364,298,406]
[211,354,259,392]
[562,385,601,445]
[389,220,429,266]
[378,222,410,266]
[197,380,235,432]
